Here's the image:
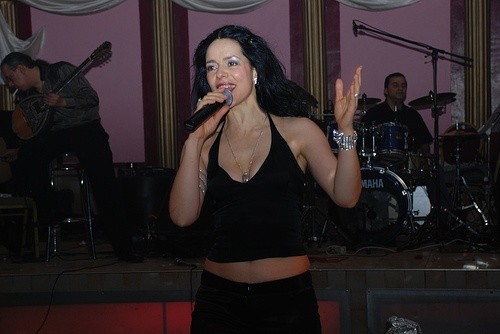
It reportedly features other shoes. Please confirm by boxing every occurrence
[113,242,145,265]
[24,239,49,264]
[438,239,467,254]
[395,232,412,244]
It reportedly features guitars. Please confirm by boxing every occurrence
[12,41,112,145]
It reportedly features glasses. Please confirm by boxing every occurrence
[5,74,12,81]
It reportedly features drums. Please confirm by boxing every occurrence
[358,149,377,167]
[374,122,408,166]
[321,121,361,152]
[331,164,411,247]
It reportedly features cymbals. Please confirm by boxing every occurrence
[438,129,477,136]
[408,92,457,106]
[358,98,381,106]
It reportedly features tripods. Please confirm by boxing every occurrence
[358,24,497,251]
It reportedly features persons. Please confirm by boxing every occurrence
[361,72,433,153]
[167,24,363,334]
[0,52,144,264]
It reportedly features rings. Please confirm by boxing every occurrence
[354,92,361,97]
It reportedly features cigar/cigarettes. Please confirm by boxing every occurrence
[12,89,19,96]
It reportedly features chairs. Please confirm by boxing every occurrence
[0,139,39,259]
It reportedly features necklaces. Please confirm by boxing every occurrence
[223,114,267,183]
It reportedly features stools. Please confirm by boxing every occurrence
[45,153,97,262]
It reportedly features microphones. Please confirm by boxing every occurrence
[353,20,358,36]
[173,258,199,267]
[182,88,233,133]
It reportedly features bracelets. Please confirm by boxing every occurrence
[335,131,358,152]
